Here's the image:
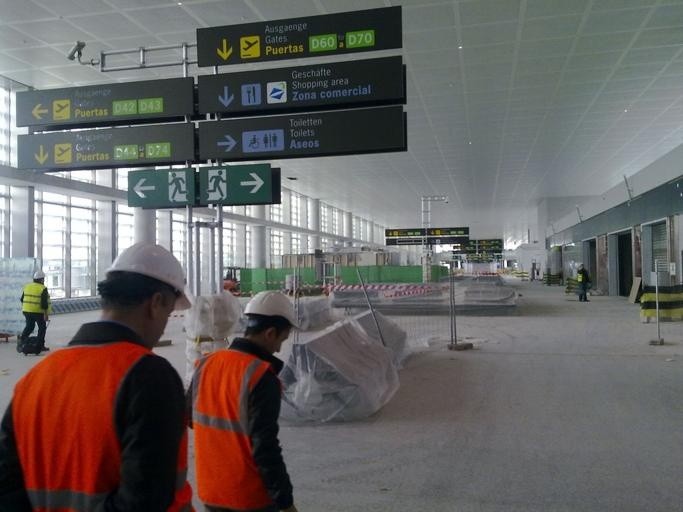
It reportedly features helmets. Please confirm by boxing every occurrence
[105,240,191,311]
[242,290,303,333]
[33,271,45,279]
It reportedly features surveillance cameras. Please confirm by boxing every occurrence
[66,41,98,65]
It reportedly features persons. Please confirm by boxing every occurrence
[0,241,198,511]
[18,270,51,351]
[576,262,592,302]
[185,290,297,512]
